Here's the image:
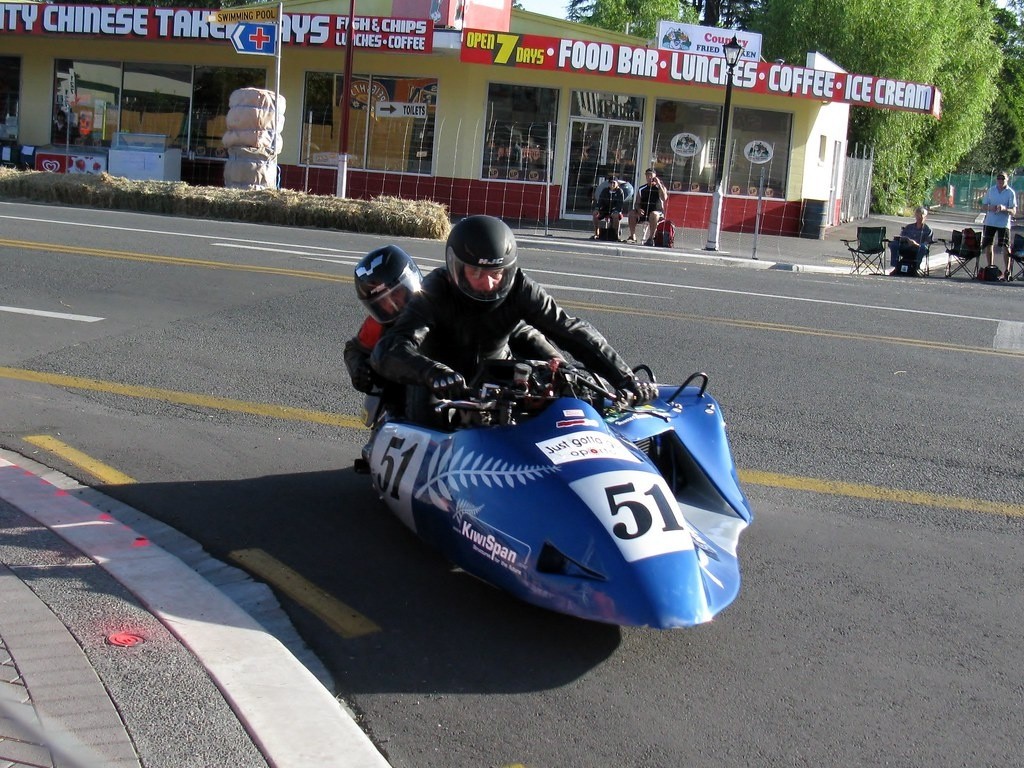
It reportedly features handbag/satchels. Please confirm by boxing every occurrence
[984,265,1000,281]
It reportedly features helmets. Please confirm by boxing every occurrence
[445,214,517,310]
[355,244,425,327]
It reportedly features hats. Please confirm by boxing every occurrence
[645,168,656,174]
[609,176,618,183]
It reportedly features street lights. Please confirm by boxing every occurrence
[700,34,744,251]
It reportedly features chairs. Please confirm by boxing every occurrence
[1006,233,1024,282]
[900,226,937,277]
[937,230,982,279]
[840,226,890,275]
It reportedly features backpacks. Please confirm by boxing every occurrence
[655,221,675,248]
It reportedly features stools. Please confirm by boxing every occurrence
[639,216,666,246]
[604,213,622,239]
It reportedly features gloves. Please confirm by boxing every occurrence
[427,366,468,400]
[617,379,659,407]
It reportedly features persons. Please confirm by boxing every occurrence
[53,111,71,140]
[628,168,667,246]
[375,214,659,424]
[79,113,91,138]
[981,171,1017,281]
[344,244,424,414]
[591,176,624,240]
[889,207,934,276]
[588,180,634,200]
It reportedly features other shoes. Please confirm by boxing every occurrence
[890,268,901,275]
[644,238,654,246]
